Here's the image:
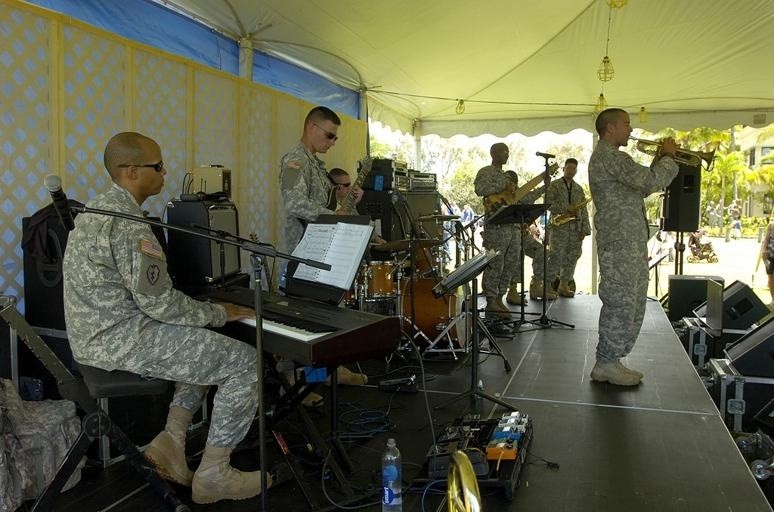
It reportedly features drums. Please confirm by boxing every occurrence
[413,247,439,275]
[391,277,471,349]
[342,259,396,299]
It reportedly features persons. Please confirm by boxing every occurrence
[327,167,388,247]
[279,108,367,406]
[732,215,744,241]
[62,130,273,503]
[473,142,587,318]
[586,109,677,389]
[443,201,473,231]
[760,222,774,309]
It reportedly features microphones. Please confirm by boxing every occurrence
[536,152,555,158]
[44,174,75,231]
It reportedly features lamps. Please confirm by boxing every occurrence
[367,0,647,123]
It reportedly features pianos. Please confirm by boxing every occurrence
[189,284,404,368]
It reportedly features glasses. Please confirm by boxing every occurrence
[337,183,350,187]
[314,124,337,141]
[119,160,163,171]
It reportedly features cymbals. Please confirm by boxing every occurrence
[417,213,460,222]
[374,238,445,253]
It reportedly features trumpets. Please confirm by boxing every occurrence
[628,137,716,171]
[550,198,591,226]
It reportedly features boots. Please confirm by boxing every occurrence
[145,406,195,486]
[486,279,574,319]
[192,447,273,504]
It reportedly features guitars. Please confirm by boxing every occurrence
[333,159,375,298]
[483,163,559,217]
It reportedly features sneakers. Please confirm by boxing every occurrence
[325,366,367,386]
[280,386,323,407]
[591,361,643,386]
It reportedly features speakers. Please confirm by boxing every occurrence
[659,162,701,232]
[22,217,73,399]
[722,315,774,377]
[668,275,724,320]
[167,200,241,284]
[356,190,443,249]
[692,279,771,331]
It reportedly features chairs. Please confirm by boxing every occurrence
[0,294,191,512]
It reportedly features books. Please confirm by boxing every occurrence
[292,222,373,291]
[488,205,508,221]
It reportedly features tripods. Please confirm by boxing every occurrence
[504,218,549,335]
[531,160,575,328]
[417,241,512,372]
[433,278,518,414]
[287,368,374,475]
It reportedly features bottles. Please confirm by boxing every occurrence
[378,435,403,507]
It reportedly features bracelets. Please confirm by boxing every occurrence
[656,152,675,160]
